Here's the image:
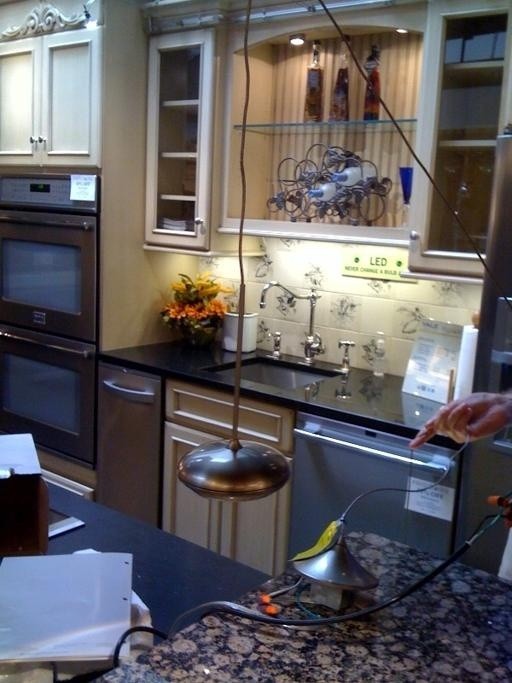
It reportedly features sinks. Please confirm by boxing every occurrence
[199,356,343,391]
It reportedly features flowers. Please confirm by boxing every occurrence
[160,271,227,340]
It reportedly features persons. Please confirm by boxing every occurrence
[407,388,512,450]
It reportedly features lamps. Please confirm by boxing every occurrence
[397,26,410,40]
[177,1,290,504]
[283,23,312,50]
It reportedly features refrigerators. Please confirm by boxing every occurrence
[95,363,164,529]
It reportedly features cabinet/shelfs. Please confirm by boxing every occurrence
[143,15,221,250]
[0,1,147,167]
[160,379,294,579]
[410,1,512,278]
[221,10,412,250]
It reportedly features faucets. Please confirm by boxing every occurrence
[259,282,311,308]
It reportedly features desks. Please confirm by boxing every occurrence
[0,473,273,683]
[92,530,512,683]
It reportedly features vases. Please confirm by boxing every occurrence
[178,324,216,356]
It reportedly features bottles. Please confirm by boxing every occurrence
[303,35,383,123]
[308,164,373,201]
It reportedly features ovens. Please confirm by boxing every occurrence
[2,174,97,472]
